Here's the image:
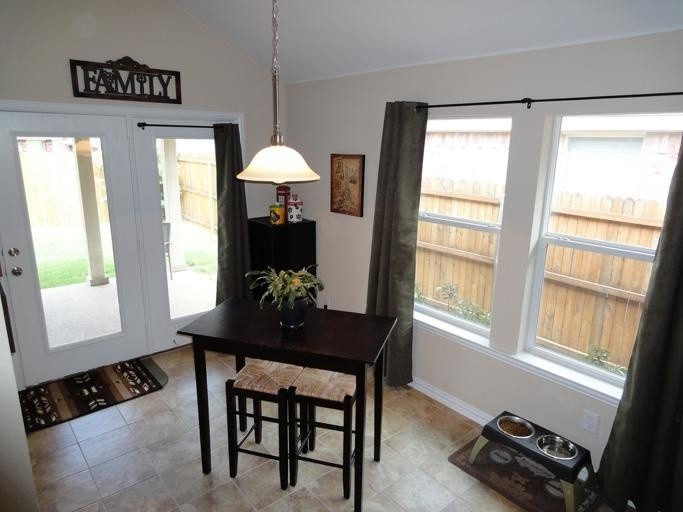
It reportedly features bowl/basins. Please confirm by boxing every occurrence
[496,415,534,439]
[535,434,578,461]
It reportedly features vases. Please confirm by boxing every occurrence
[258,294,311,338]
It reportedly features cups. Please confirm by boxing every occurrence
[268,205,284,225]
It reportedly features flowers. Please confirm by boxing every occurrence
[243,263,323,310]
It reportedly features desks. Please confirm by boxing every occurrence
[466,408,598,512]
[174,288,401,512]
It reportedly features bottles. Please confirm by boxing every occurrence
[285,194,302,224]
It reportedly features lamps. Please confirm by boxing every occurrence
[234,0,322,184]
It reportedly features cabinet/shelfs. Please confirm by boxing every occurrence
[242,214,318,307]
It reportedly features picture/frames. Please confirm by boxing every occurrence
[329,151,365,221]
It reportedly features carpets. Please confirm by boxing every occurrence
[15,350,171,433]
[445,425,591,512]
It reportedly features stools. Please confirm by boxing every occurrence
[289,358,364,501]
[222,357,307,492]
